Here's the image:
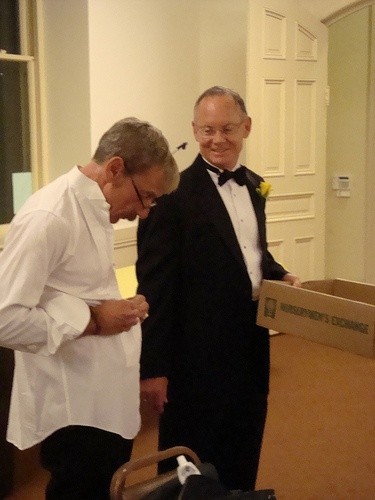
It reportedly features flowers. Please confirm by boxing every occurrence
[256,182,273,199]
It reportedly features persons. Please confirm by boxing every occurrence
[135,86,302,491]
[0,117,180,500]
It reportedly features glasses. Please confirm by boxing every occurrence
[123,162,157,209]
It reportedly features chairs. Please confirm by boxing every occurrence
[109,446,218,500]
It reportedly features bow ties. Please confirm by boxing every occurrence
[203,160,246,186]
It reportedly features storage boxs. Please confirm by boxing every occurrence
[256,276,375,361]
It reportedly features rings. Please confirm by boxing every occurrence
[144,398,148,402]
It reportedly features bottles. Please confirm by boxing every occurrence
[177,455,201,485]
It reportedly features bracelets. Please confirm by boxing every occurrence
[89,304,104,335]
